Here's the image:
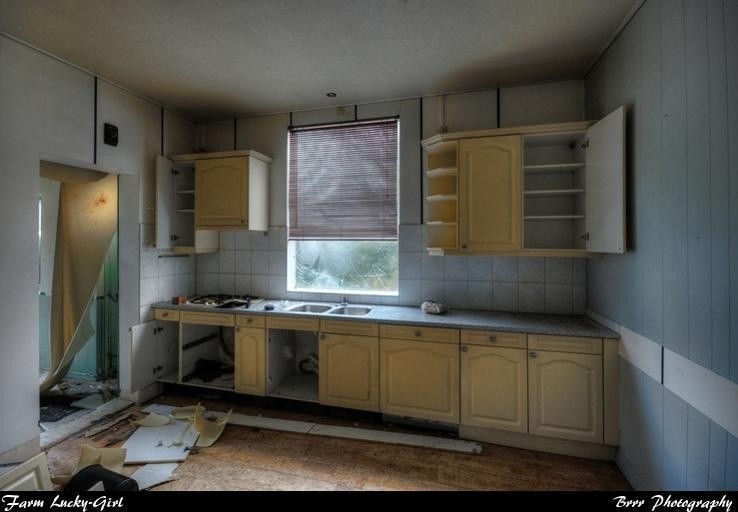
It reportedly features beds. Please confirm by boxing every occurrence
[417,104,630,257]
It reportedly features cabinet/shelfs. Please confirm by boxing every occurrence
[130,307,621,459]
[153,149,272,257]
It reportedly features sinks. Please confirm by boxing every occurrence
[329,305,373,318]
[288,301,333,314]
